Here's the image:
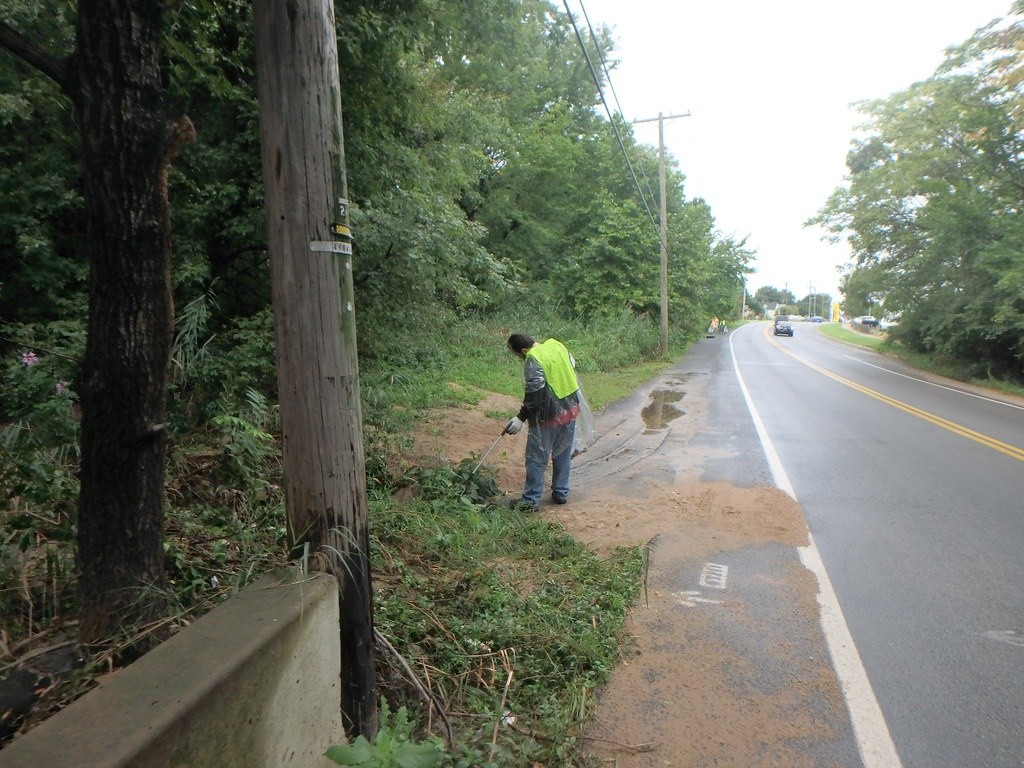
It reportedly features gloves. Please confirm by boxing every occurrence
[506,416,523,435]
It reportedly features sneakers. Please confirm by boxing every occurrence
[552,492,566,503]
[510,498,539,513]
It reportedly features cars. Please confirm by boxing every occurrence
[774,320,794,337]
[775,315,787,325]
[812,316,822,323]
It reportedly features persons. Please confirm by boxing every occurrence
[711,317,727,333]
[506,333,581,511]
